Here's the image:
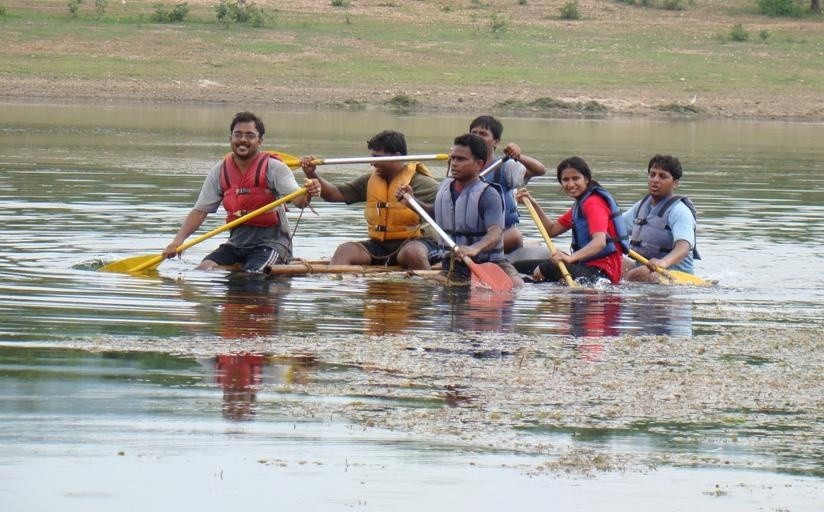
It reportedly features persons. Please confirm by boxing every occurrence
[395,133,525,293]
[515,157,624,289]
[300,131,441,271]
[162,112,322,281]
[469,115,545,253]
[622,153,701,286]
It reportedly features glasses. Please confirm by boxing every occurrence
[231,132,260,140]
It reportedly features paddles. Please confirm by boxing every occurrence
[402,193,513,294]
[522,196,581,288]
[479,151,518,176]
[95,180,313,278]
[628,249,712,287]
[223,150,449,171]
